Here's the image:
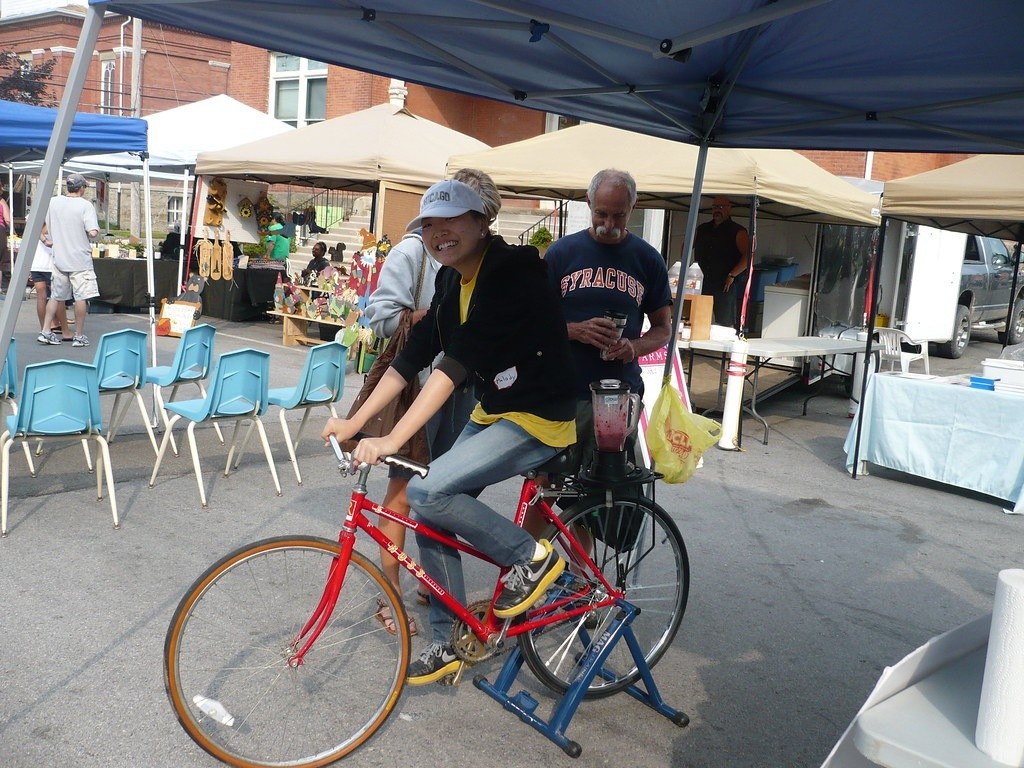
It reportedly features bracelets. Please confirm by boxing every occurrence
[729,273,736,278]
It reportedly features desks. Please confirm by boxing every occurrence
[843,371,1024,514]
[689,335,885,445]
[91,257,287,322]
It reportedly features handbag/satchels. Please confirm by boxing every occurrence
[339,310,431,465]
[647,375,724,483]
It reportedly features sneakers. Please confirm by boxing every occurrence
[37,333,61,344]
[493,539,565,618]
[71,332,90,347]
[404,642,473,685]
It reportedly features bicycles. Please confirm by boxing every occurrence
[162,421,691,768]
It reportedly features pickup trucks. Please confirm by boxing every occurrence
[934,234,1024,359]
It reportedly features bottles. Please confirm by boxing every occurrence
[600,313,628,361]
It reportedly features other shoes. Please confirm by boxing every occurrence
[66,318,75,324]
[577,603,601,630]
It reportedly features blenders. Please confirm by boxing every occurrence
[588,378,641,485]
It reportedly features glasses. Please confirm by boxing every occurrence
[712,204,728,209]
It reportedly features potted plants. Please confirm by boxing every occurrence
[528,227,553,259]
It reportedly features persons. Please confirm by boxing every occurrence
[66,318,74,324]
[364,169,501,635]
[681,195,749,361]
[266,212,292,298]
[31,220,62,334]
[544,163,673,630]
[322,178,578,684]
[0,179,18,295]
[37,173,100,347]
[302,242,330,325]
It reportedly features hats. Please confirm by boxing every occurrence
[406,179,486,232]
[66,174,90,187]
[320,242,328,252]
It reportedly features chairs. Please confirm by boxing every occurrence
[0,358,121,538]
[35,328,159,474]
[233,342,349,485]
[0,337,36,477]
[877,327,929,374]
[148,347,282,509]
[110,323,224,458]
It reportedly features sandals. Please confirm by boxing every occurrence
[62,338,73,341]
[415,588,430,606]
[375,598,417,636]
[51,325,62,334]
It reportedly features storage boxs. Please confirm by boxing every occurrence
[744,253,799,332]
[981,358,1024,388]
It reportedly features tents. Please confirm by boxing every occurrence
[185,102,494,283]
[89,0,1024,592]
[0,93,298,327]
[0,100,159,428]
[446,121,880,449]
[853,154,1024,482]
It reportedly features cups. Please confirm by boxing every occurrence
[100,251,105,258]
